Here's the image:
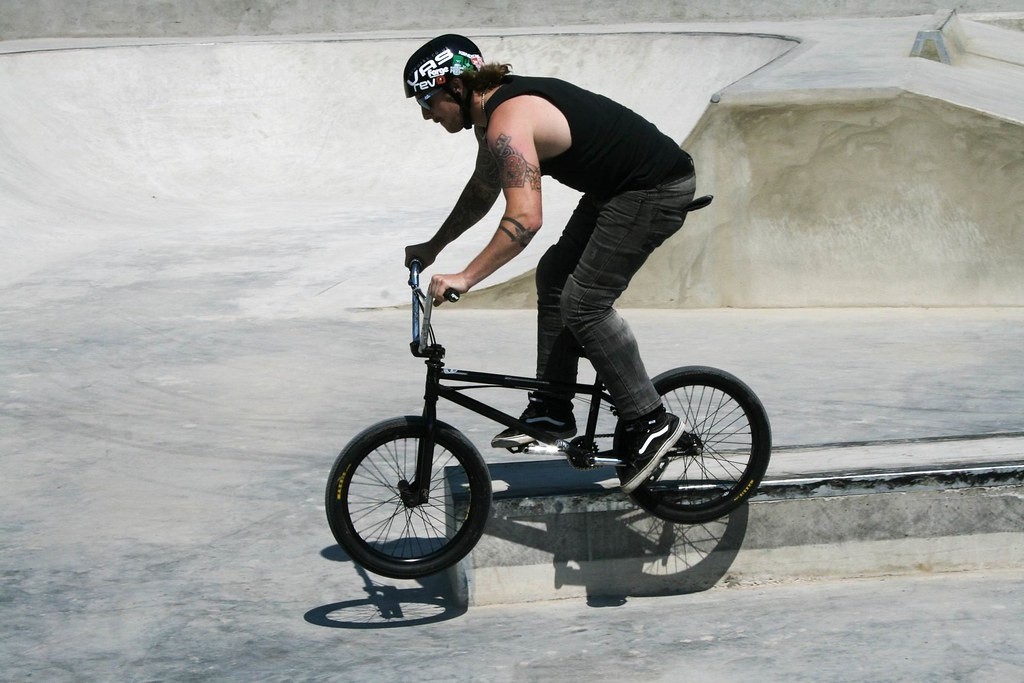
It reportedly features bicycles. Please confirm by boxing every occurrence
[324,256,774,579]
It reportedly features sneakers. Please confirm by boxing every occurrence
[619,407,685,494]
[491,391,577,447]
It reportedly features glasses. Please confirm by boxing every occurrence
[415,87,443,110]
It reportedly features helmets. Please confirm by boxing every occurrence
[404,34,484,98]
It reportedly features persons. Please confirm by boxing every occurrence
[393,32,702,496]
